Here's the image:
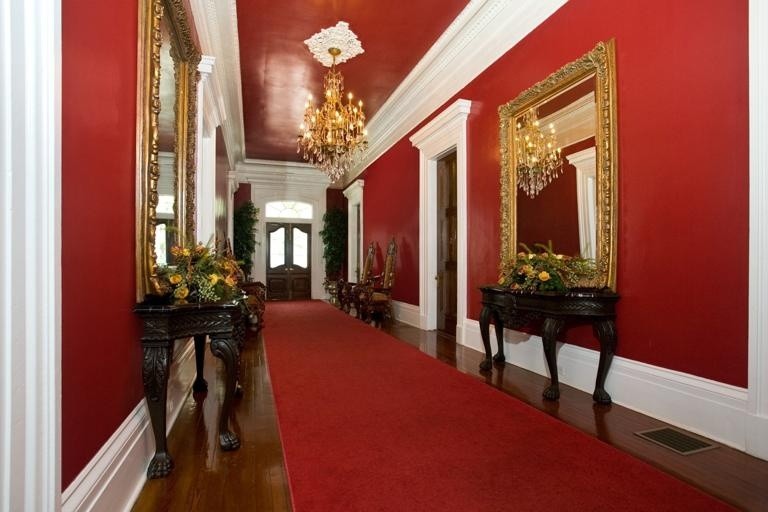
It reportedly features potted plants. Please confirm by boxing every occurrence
[320,207,349,307]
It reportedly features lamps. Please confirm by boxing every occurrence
[295,20,369,185]
[512,108,564,198]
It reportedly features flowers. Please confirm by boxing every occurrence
[500,256,567,295]
[150,243,244,304]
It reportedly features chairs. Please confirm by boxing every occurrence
[223,238,267,337]
[340,232,399,325]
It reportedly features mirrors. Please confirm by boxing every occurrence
[497,37,616,293]
[134,1,203,303]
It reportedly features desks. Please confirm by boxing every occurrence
[477,283,621,404]
[138,290,249,479]
